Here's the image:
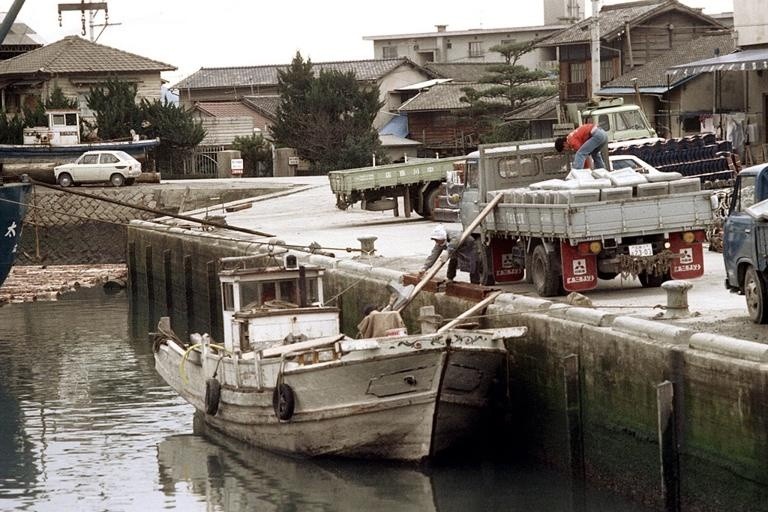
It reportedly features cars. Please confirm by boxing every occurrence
[52,148,144,188]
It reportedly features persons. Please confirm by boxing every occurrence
[555,123,611,172]
[418,228,480,285]
[362,294,398,316]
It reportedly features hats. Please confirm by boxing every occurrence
[363,303,379,316]
[430,224,447,241]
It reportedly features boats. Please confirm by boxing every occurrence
[0,163,33,292]
[144,245,509,467]
[155,415,439,512]
[0,107,164,164]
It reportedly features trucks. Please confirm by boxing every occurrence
[328,101,659,221]
[721,160,767,329]
[441,132,716,302]
